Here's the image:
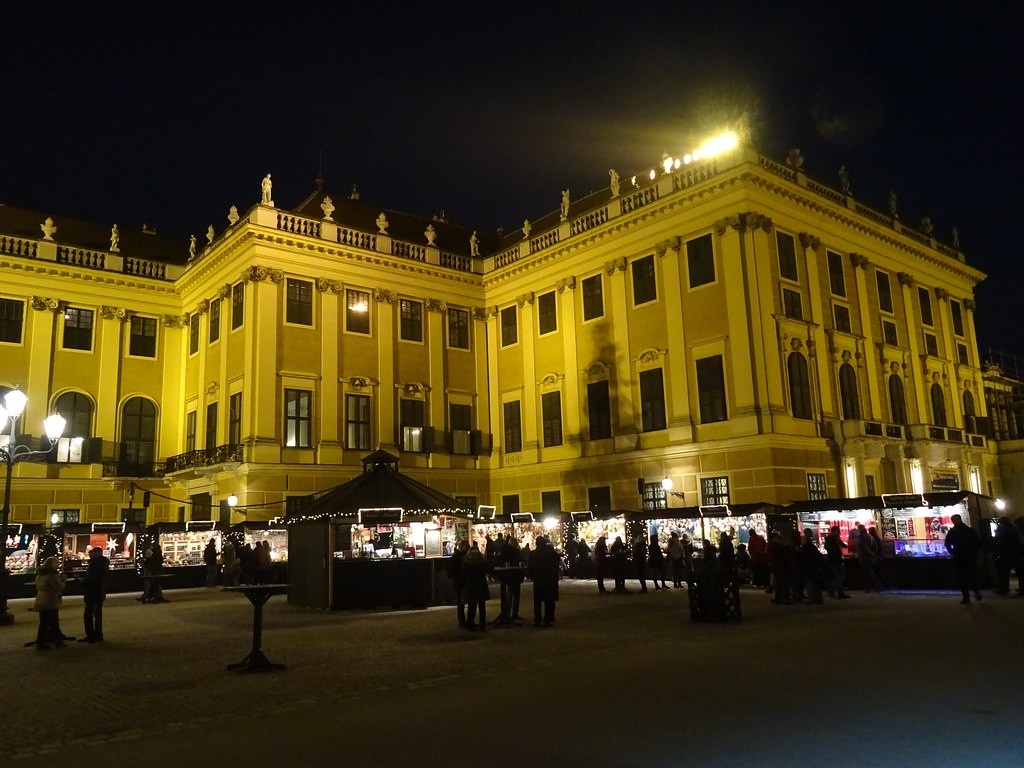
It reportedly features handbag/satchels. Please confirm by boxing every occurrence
[767,563,774,572]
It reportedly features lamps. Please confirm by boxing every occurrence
[662,476,685,501]
[228,492,246,516]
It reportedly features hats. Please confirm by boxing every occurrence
[671,532,678,539]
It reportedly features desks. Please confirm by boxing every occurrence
[488,566,530,629]
[219,583,295,672]
[139,573,175,605]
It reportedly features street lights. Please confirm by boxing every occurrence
[0,387,67,626]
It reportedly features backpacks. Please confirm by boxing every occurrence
[446,557,457,579]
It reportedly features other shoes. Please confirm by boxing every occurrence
[470,627,475,631]
[600,588,609,593]
[511,615,523,620]
[638,587,647,593]
[544,623,553,628]
[655,585,662,590]
[77,636,103,643]
[661,585,670,589]
[960,598,970,604]
[56,641,69,647]
[975,594,981,601]
[480,624,486,632]
[674,584,678,588]
[678,584,683,588]
[38,644,53,651]
[534,623,542,627]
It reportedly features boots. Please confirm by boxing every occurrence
[765,585,773,594]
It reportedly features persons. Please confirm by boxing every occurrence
[470,231,481,257]
[765,521,823,605]
[78,547,110,643]
[486,533,556,624]
[662,531,698,588]
[592,537,609,593]
[446,541,490,631]
[735,528,768,589]
[262,173,272,202]
[137,542,163,601]
[35,557,66,650]
[888,190,898,217]
[824,521,884,597]
[189,235,196,256]
[609,169,620,199]
[632,534,647,592]
[204,538,221,587]
[838,165,852,195]
[560,190,569,217]
[953,227,959,247]
[611,537,624,587]
[931,515,1024,604]
[220,540,272,587]
[694,532,735,561]
[528,536,560,628]
[111,224,119,247]
[565,533,591,579]
[648,534,670,589]
